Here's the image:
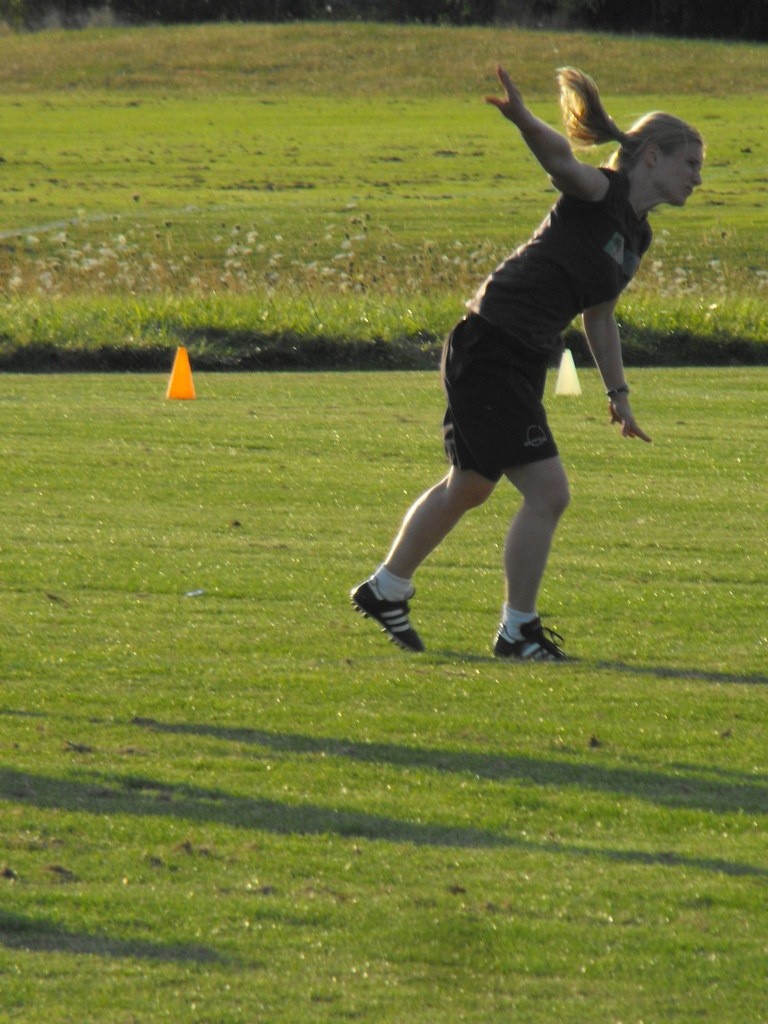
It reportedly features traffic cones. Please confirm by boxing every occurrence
[165,347,198,400]
[555,349,581,397]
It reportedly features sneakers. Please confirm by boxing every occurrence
[351,576,425,654]
[492,618,567,663]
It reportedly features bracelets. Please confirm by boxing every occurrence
[607,386,629,398]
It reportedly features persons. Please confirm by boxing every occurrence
[351,66,703,662]
